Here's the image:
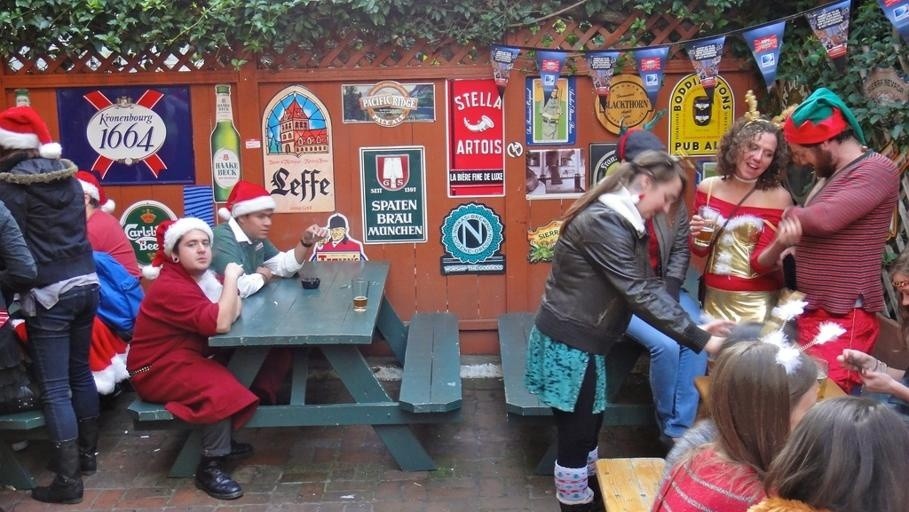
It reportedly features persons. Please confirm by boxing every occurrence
[0,170,146,427]
[611,126,710,453]
[310,212,370,263]
[523,148,733,511]
[209,179,331,299]
[127,216,260,500]
[686,117,804,334]
[836,244,909,405]
[747,396,909,512]
[0,105,101,505]
[650,334,820,511]
[780,87,901,392]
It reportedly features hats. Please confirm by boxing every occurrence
[0,106,63,159]
[142,217,214,280]
[615,131,667,161]
[218,181,276,220]
[74,171,116,214]
[328,216,345,229]
[784,87,868,153]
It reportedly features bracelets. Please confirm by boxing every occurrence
[300,236,314,249]
[870,357,887,375]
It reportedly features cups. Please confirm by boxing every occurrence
[351,278,370,313]
[574,173,582,193]
[811,358,830,404]
[692,204,719,248]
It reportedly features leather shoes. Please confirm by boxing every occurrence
[221,440,253,461]
[195,462,244,499]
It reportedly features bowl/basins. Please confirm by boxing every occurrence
[296,270,324,291]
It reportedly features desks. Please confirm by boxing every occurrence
[165,256,443,478]
[532,345,851,478]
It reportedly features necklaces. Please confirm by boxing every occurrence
[732,172,759,186]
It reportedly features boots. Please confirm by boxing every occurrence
[32,437,83,503]
[48,415,98,475]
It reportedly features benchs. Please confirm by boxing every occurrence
[1,400,51,495]
[123,395,236,485]
[590,457,678,512]
[495,308,613,481]
[381,304,480,473]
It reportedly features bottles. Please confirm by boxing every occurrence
[211,79,242,205]
[14,88,31,109]
